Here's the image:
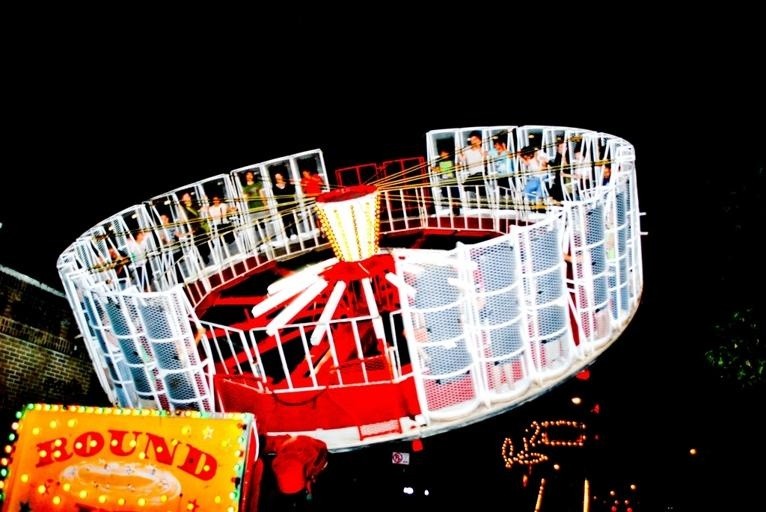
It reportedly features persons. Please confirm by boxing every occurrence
[433,131,612,213]
[94,170,326,292]
